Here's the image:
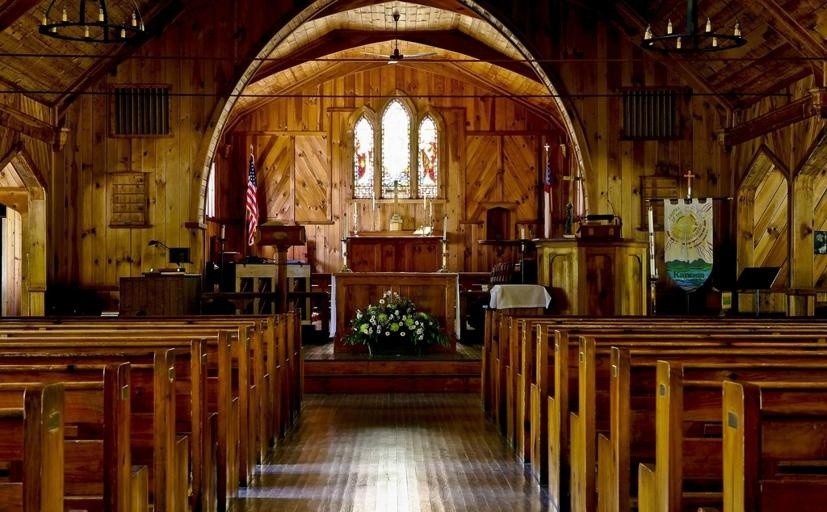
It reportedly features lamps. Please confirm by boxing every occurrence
[639,0,747,55]
[40,0,145,44]
[148,240,190,264]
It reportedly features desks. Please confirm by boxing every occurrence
[119,274,202,317]
[345,236,443,272]
[333,273,458,354]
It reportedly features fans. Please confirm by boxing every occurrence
[363,15,437,64]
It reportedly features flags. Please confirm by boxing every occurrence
[544,152,553,239]
[245,153,259,246]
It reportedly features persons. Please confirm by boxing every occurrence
[489,245,521,284]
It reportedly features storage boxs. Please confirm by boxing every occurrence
[493,284,548,316]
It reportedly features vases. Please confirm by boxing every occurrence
[368,339,417,357]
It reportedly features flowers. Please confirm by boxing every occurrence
[340,289,452,349]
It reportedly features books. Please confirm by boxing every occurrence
[100,304,120,317]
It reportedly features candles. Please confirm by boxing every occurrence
[443,215,447,273]
[354,201,358,235]
[520,227,525,283]
[423,191,427,226]
[430,199,433,236]
[372,191,375,232]
[340,213,354,272]
[221,223,225,270]
